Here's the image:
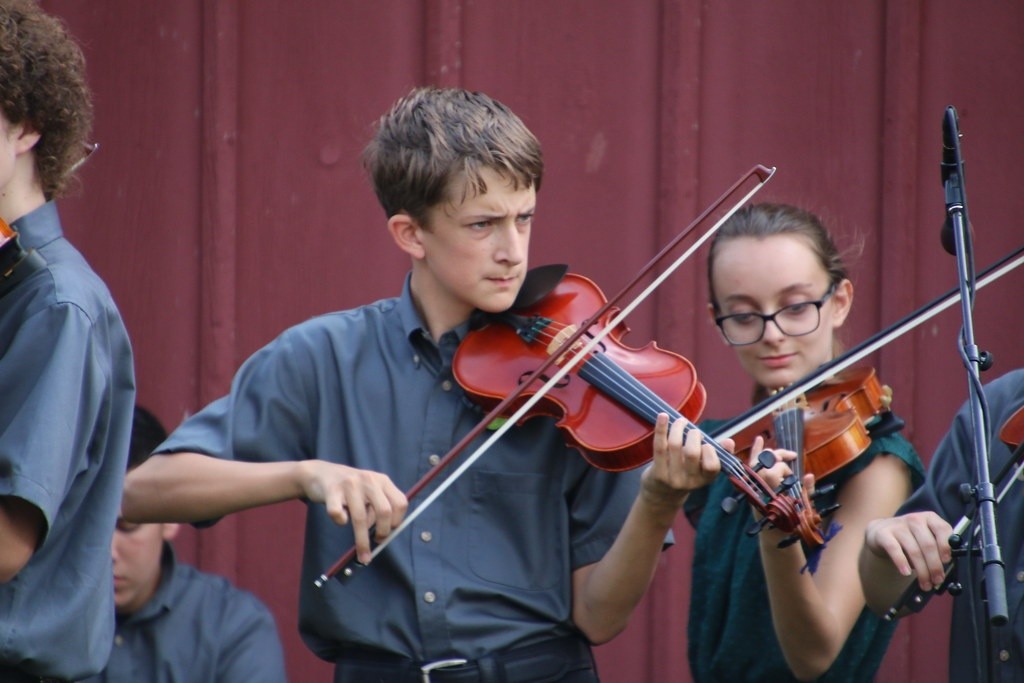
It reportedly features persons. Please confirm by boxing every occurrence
[2,4,141,683]
[123,87,738,683]
[857,374,1019,683]
[689,201,922,683]
[111,403,286,682]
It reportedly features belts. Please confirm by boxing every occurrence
[334,635,593,683]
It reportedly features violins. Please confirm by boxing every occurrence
[996,403,1024,474]
[721,365,886,553]
[445,272,806,538]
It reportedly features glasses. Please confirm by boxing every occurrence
[714,275,843,346]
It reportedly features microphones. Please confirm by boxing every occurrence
[941,105,971,255]
